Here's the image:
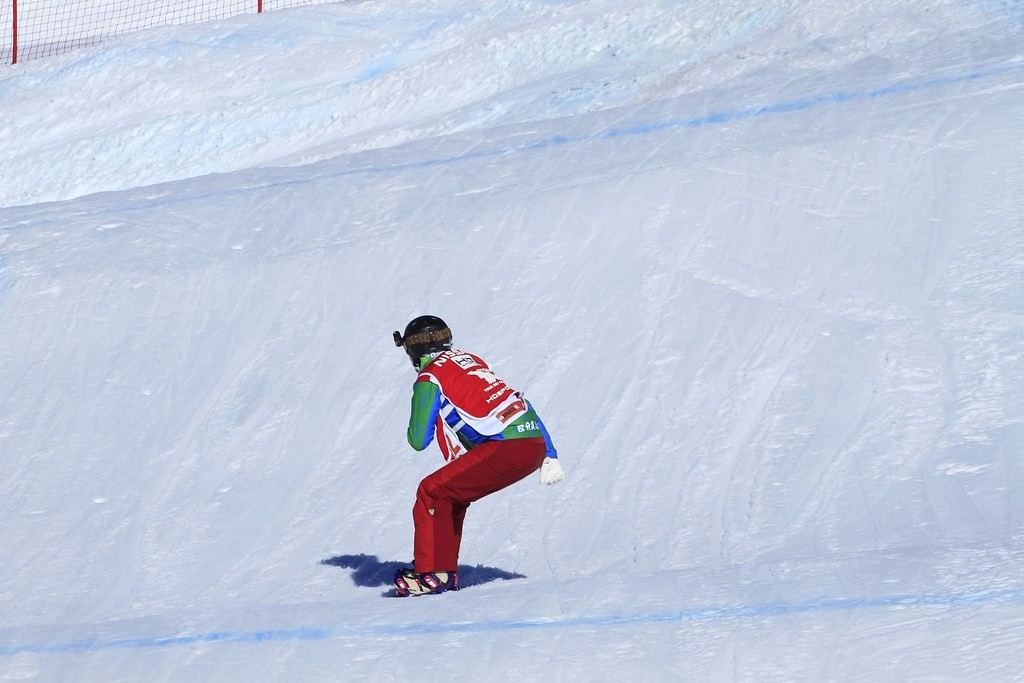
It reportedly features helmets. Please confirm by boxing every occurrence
[393,315,452,367]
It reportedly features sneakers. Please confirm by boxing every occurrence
[392,564,460,599]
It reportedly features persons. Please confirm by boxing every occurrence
[394,315,564,595]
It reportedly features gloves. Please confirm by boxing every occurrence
[540,457,565,484]
